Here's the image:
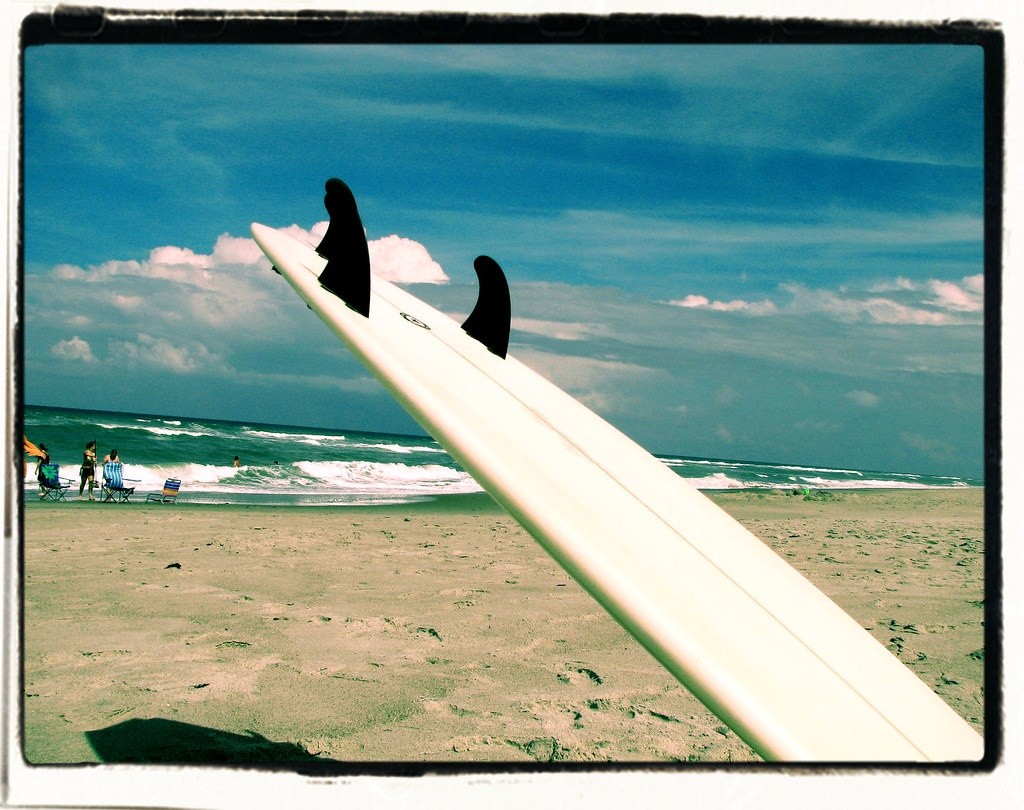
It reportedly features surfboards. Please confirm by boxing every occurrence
[247,220,985,764]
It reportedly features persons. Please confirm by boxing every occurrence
[234,455,240,467]
[78,442,97,500]
[35,443,49,496]
[273,460,280,466]
[102,449,120,502]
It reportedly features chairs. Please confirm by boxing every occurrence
[100,462,135,504]
[146,478,182,505]
[38,463,76,502]
